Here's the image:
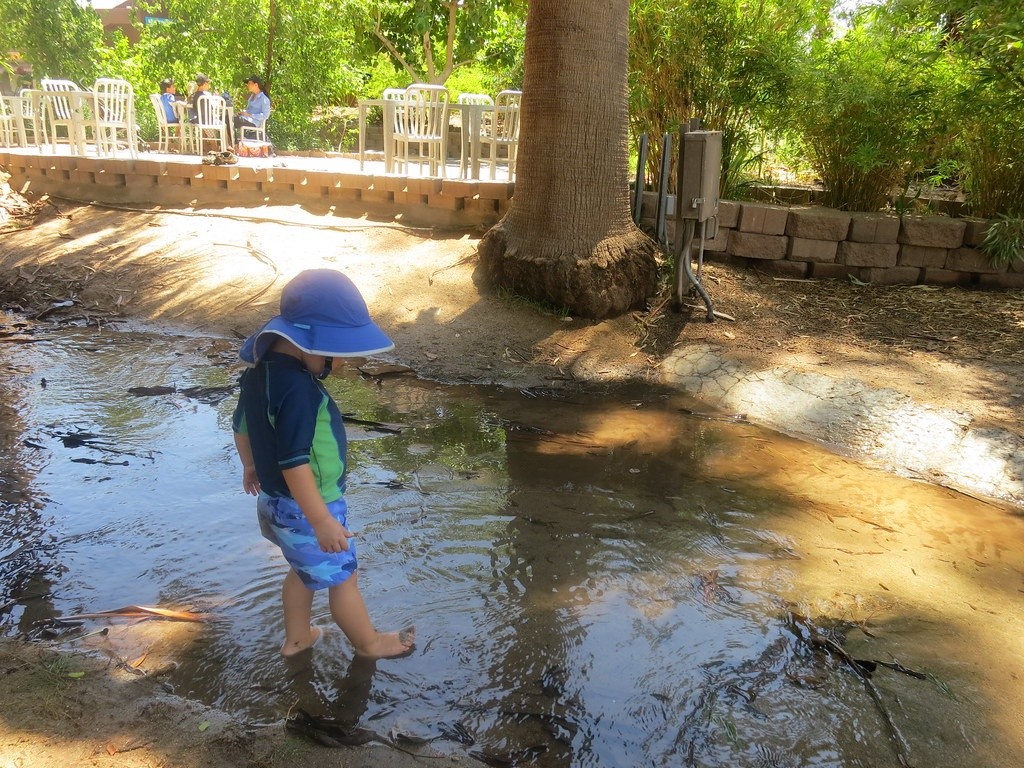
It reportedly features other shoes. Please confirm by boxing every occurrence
[202,150,238,166]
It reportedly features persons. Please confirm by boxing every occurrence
[230,268,416,658]
[159,74,272,152]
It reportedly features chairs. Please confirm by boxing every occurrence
[0,77,523,180]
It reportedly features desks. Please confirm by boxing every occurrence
[359,99,517,180]
[31,90,138,155]
[178,104,234,155]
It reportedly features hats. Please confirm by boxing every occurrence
[238,269,396,365]
[242,75,262,84]
